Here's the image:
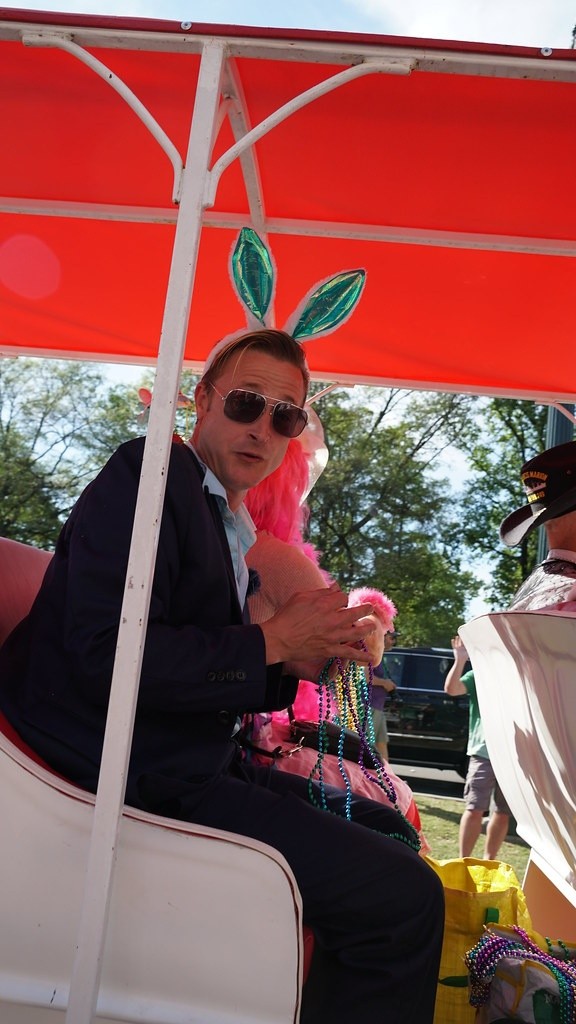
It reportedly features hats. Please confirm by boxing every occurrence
[384,629,401,638]
[500,441,576,547]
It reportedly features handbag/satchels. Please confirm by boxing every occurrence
[426,853,562,1024]
[291,720,385,768]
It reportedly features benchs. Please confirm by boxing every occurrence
[0,537,313,1024]
[458,611,576,941]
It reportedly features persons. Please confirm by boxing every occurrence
[0,329,444,1024]
[500,441,576,613]
[364,627,399,762]
[444,636,510,859]
[244,437,430,858]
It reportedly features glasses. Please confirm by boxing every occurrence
[209,380,309,438]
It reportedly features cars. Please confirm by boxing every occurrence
[378,646,473,781]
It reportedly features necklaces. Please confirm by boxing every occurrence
[465,922,576,1024]
[305,640,422,852]
